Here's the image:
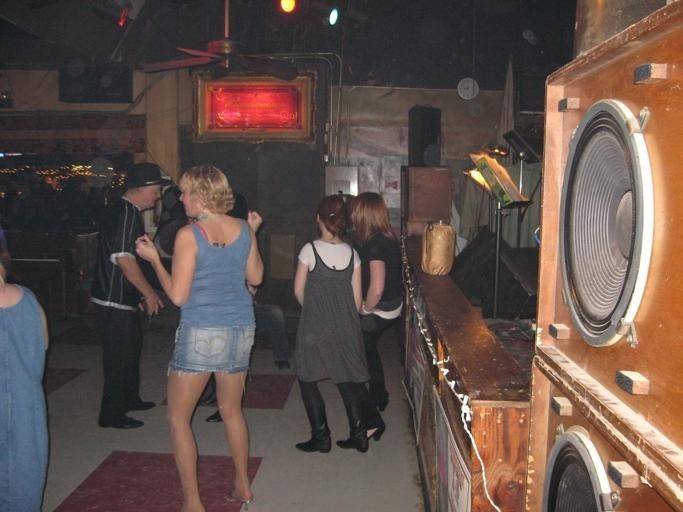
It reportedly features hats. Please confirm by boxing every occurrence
[119,162,169,190]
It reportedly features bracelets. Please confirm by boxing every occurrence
[141,290,155,300]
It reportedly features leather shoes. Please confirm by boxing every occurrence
[125,401,155,412]
[206,410,222,422]
[336,437,369,452]
[295,439,331,453]
[111,415,144,428]
[198,391,217,406]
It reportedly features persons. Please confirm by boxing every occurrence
[90,161,166,430]
[1,228,51,512]
[134,160,265,512]
[292,191,405,453]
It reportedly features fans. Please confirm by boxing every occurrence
[141,0,297,81]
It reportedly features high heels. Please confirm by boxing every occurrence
[225,492,254,512]
[375,397,389,411]
[368,425,385,441]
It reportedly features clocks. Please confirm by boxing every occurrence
[456,77,480,101]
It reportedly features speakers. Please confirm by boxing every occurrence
[533,0,683,494]
[524,355,683,512]
[58,61,134,104]
[407,104,442,167]
